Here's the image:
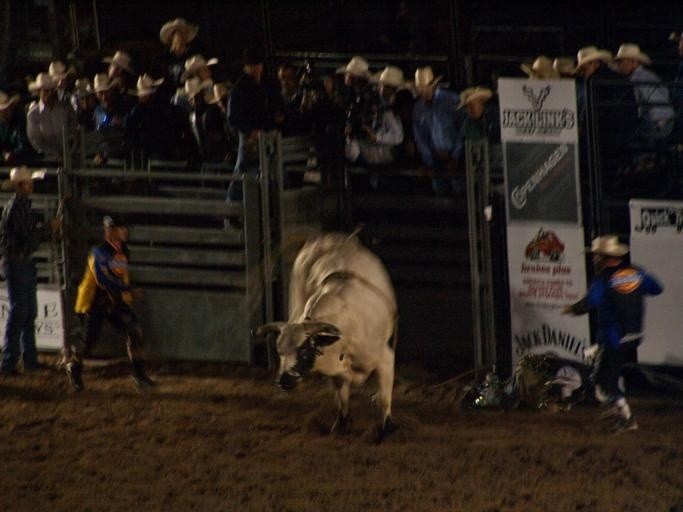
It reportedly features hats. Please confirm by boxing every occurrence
[1,166,47,189]
[0,17,271,111]
[589,235,630,257]
[104,213,135,227]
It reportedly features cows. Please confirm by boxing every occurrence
[255,233,400,438]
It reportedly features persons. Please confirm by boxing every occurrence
[0,165,61,373]
[63,212,153,392]
[0,17,683,207]
[564,232,665,434]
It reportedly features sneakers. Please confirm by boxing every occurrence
[603,414,638,433]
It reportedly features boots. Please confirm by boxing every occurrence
[65,361,84,392]
[129,358,154,386]
